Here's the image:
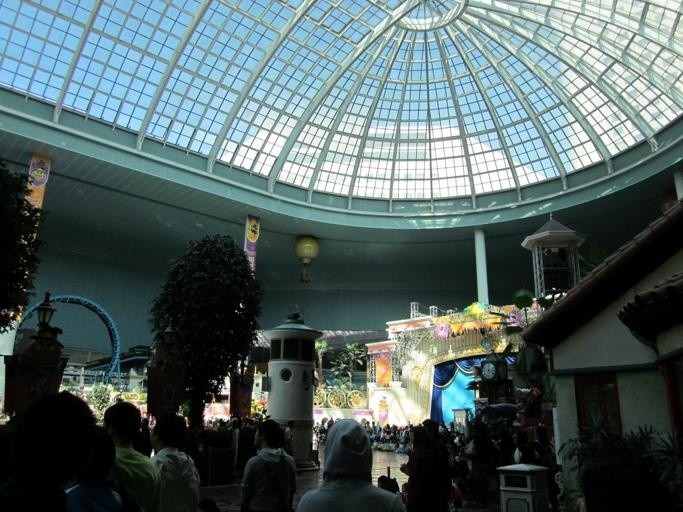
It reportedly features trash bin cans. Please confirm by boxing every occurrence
[496,464,549,512]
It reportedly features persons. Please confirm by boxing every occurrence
[0,408,564,512]
